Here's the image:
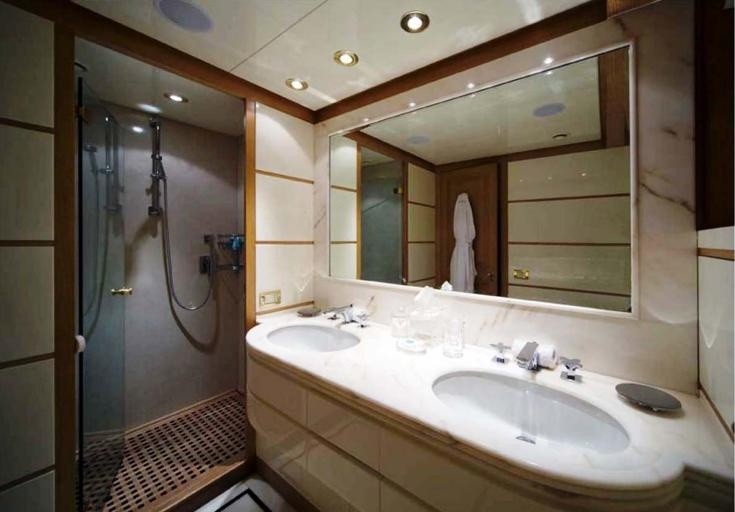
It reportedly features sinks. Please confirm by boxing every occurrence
[267,322,360,352]
[433,370,632,456]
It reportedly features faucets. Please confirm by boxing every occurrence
[331,308,350,324]
[527,350,541,371]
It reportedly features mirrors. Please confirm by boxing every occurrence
[325,40,639,322]
